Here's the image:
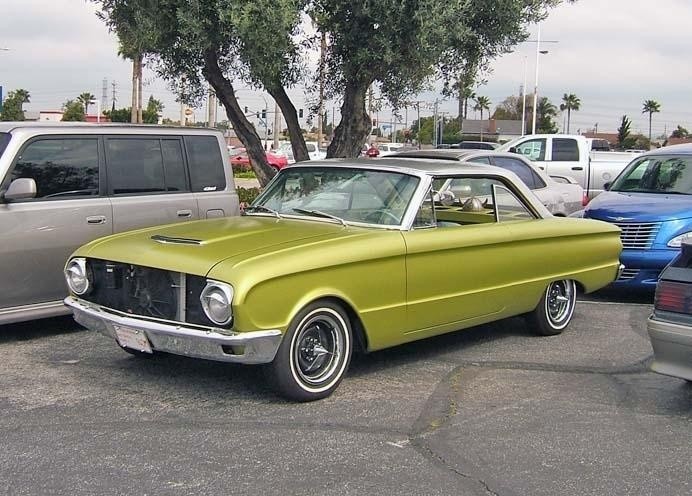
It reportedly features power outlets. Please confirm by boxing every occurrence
[493,133,644,200]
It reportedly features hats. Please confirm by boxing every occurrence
[645,234,691,383]
[581,141,691,292]
[227,139,404,175]
[437,140,500,149]
[63,157,625,403]
[283,149,589,218]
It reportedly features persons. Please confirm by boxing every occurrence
[366,142,380,158]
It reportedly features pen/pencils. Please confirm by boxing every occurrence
[2,119,242,327]
